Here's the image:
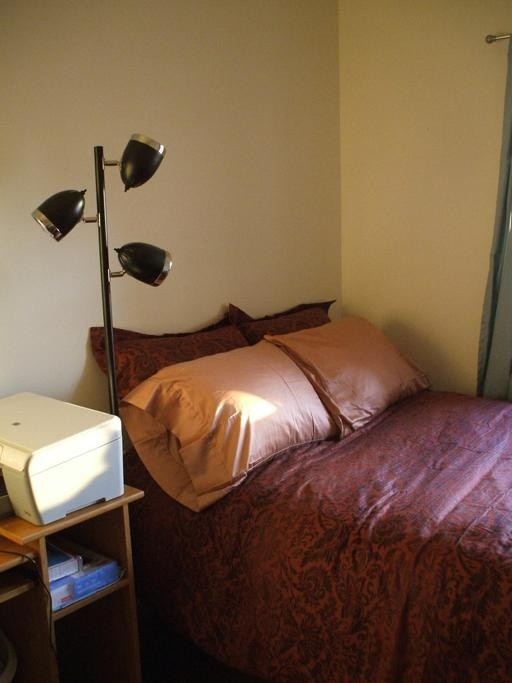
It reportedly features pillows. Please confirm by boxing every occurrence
[117,339,336,512]
[83,313,247,404]
[227,300,334,345]
[264,315,431,439]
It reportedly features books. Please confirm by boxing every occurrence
[48,534,126,613]
[45,538,83,584]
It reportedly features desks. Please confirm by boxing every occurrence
[0,483,145,682]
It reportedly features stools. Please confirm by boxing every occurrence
[0,635,19,681]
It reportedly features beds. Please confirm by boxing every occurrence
[89,299,512,678]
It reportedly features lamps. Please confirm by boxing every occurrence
[31,133,173,414]
